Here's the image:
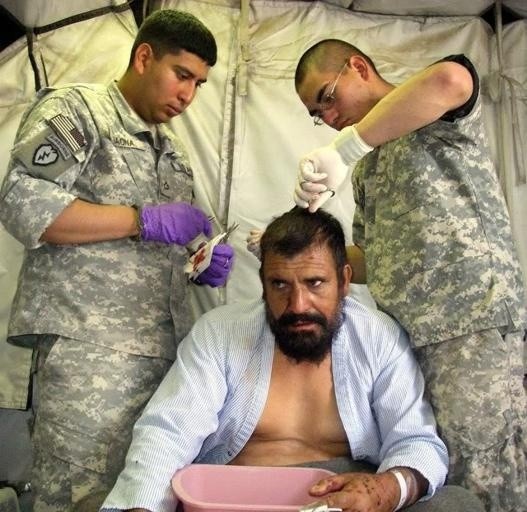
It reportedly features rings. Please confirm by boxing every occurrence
[224,258,229,267]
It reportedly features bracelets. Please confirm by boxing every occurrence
[389,468,409,512]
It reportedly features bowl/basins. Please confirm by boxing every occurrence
[171,463,340,512]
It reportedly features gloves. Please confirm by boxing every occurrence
[190,242,234,288]
[138,202,214,245]
[294,128,368,213]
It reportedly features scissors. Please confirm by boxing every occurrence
[219,222,240,267]
[290,181,336,212]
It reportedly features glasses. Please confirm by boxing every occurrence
[313,61,349,132]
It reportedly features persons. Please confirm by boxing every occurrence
[96,204,449,512]
[246,38,526,510]
[0,8,234,512]
